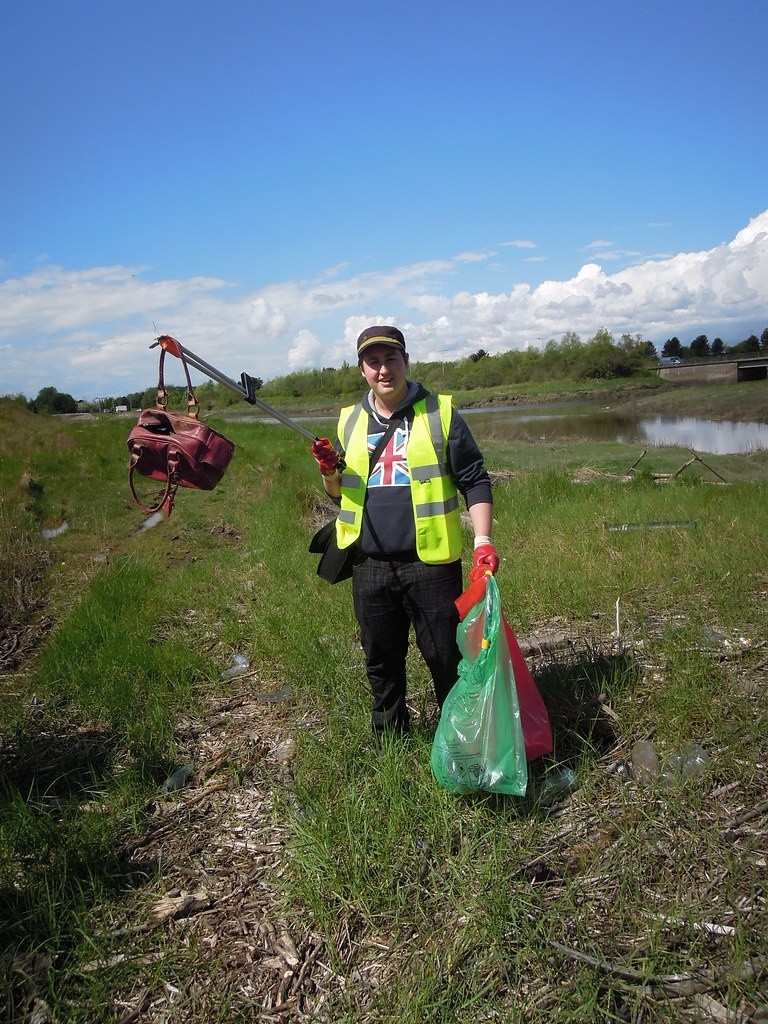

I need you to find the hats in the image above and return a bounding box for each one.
[357,326,405,360]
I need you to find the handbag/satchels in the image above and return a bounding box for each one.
[309,518,362,585]
[126,335,235,513]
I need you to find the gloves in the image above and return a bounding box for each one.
[472,535,500,575]
[311,438,341,482]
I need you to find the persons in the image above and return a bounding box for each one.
[312,326,498,752]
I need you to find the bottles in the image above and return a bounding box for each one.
[219,652,253,681]
[532,769,580,801]
[629,742,664,789]
[273,739,297,766]
[657,744,710,787]
